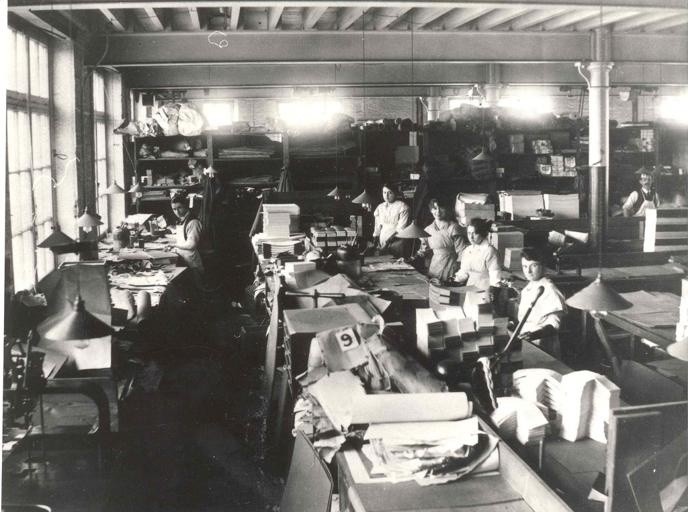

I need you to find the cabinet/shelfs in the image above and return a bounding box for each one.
[421,126,661,206]
[288,123,422,239]
[129,127,287,240]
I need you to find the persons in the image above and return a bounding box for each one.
[514,249,570,362]
[371,179,410,253]
[405,194,468,285]
[621,169,662,217]
[161,191,209,277]
[449,216,509,317]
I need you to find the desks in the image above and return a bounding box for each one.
[3,201,688,511]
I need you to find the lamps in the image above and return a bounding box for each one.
[31,0,120,253]
[326,14,348,200]
[395,10,434,241]
[349,9,381,213]
[561,12,638,322]
[40,0,122,354]
